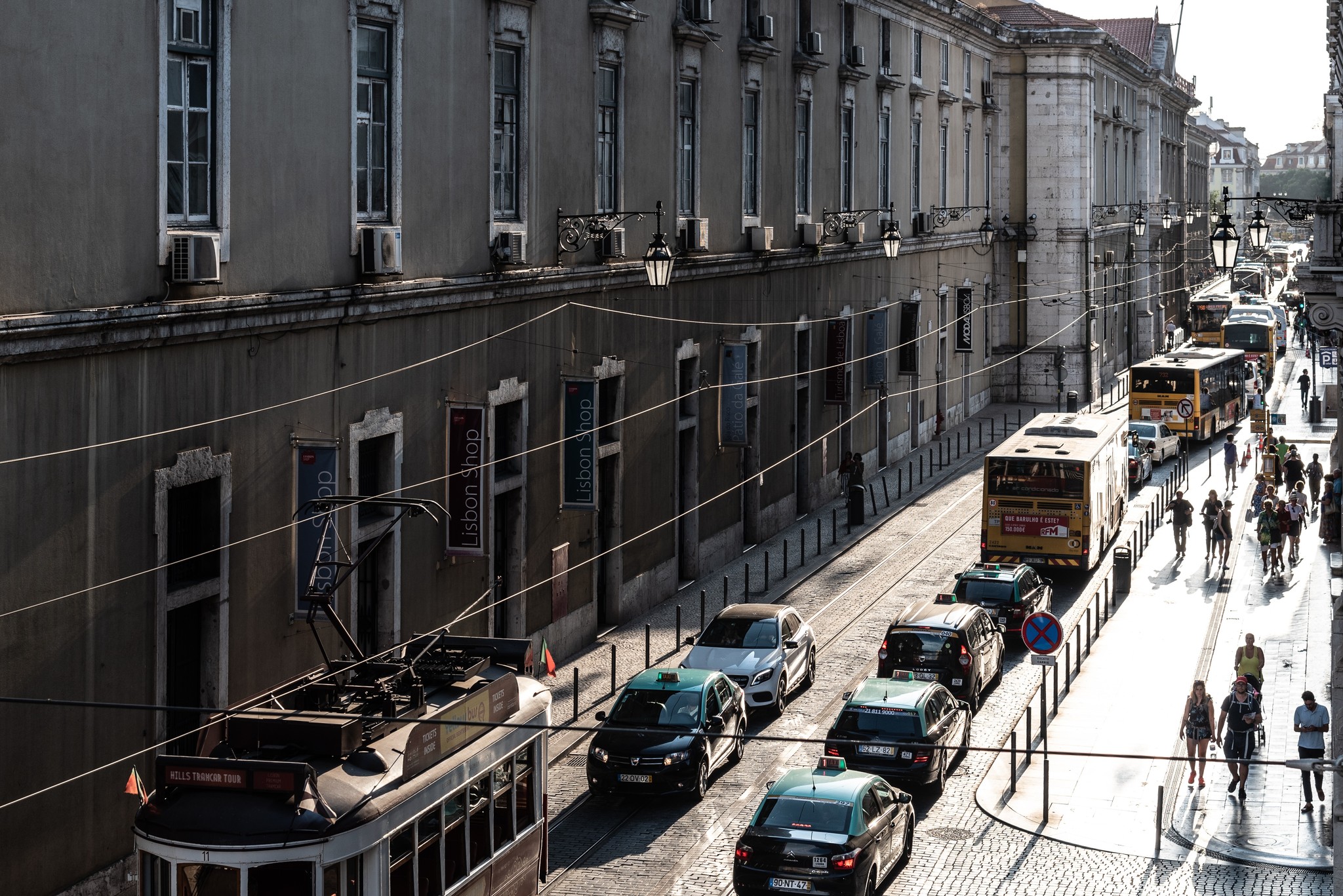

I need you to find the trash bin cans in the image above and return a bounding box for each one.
[1309,396,1321,423]
[1113,545,1131,593]
[848,485,864,524]
[1067,391,1078,413]
[1188,319,1191,335]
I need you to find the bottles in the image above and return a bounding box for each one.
[1210,741,1216,759]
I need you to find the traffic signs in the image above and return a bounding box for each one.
[1319,346,1338,368]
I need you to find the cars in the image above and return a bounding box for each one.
[1128,438,1154,488]
[823,670,972,791]
[587,668,748,799]
[678,602,817,717]
[731,756,916,896]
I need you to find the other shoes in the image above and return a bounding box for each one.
[1238,787,1246,799]
[1301,804,1314,812]
[1317,788,1325,801]
[1228,775,1240,793]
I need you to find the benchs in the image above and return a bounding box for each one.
[1331,578,1343,603]
[1330,552,1343,576]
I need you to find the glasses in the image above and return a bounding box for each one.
[1265,504,1271,507]
[1292,507,1295,513]
[1279,503,1285,506]
[1245,637,1253,641]
[1304,700,1314,707]
[1256,477,1262,479]
[1236,682,1247,687]
[1194,680,1204,685]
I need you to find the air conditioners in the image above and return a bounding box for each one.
[984,80,994,98]
[1115,106,1122,118]
[602,228,625,257]
[1094,255,1101,268]
[917,212,932,234]
[806,32,821,53]
[686,218,708,250]
[1090,305,1099,319]
[751,223,865,250]
[850,45,864,65]
[361,226,402,274]
[499,231,527,263]
[692,0,712,22]
[1106,251,1114,264]
[757,15,773,38]
[172,234,220,283]
[1130,243,1135,258]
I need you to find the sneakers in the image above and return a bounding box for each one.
[1199,777,1205,786]
[1188,770,1196,784]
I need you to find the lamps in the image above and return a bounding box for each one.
[557,200,676,292]
[1092,186,1327,273]
[930,200,995,248]
[822,201,903,261]
[1001,213,1038,251]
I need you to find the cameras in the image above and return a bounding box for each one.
[1166,519,1172,524]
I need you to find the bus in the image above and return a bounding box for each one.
[1216,304,1282,382]
[1186,291,1241,348]
[980,413,1141,573]
[1228,235,1300,327]
[134,495,551,893]
[1125,347,1254,442]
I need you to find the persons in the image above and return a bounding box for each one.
[1223,433,1239,490]
[1188,265,1215,290]
[1165,491,1194,556]
[1166,320,1176,348]
[1216,500,1235,569]
[1234,633,1264,689]
[1297,369,1310,408]
[1216,676,1263,798]
[837,451,867,503]
[1252,687,1259,700]
[1180,680,1216,786]
[1252,388,1268,423]
[1250,427,1342,574]
[1293,313,1338,348]
[1199,387,1217,412]
[1201,490,1223,559]
[1294,691,1330,812]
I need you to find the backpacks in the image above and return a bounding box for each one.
[1212,511,1231,534]
[1228,682,1253,713]
[1187,694,1211,730]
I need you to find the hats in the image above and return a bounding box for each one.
[1289,449,1298,456]
[1289,494,1299,501]
[1236,676,1247,684]
[1222,500,1235,509]
[1253,687,1260,695]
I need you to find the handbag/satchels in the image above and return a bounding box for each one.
[1202,513,1210,519]
[1184,500,1192,527]
[1251,505,1256,518]
[1269,527,1282,544]
[1245,505,1253,523]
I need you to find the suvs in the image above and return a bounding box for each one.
[871,592,1007,713]
[1126,421,1181,464]
[952,562,1053,638]
[1243,361,1264,402]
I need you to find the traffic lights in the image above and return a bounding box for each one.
[1260,394,1265,405]
[1300,320,1303,326]
[1258,354,1266,375]
[1299,295,1305,309]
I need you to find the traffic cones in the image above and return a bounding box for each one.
[1257,433,1264,450]
[1245,443,1253,459]
[1239,450,1251,468]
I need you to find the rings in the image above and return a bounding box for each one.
[1248,722,1249,722]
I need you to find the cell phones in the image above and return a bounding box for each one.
[1304,726,1310,728]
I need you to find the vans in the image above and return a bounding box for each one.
[1259,303,1290,353]
